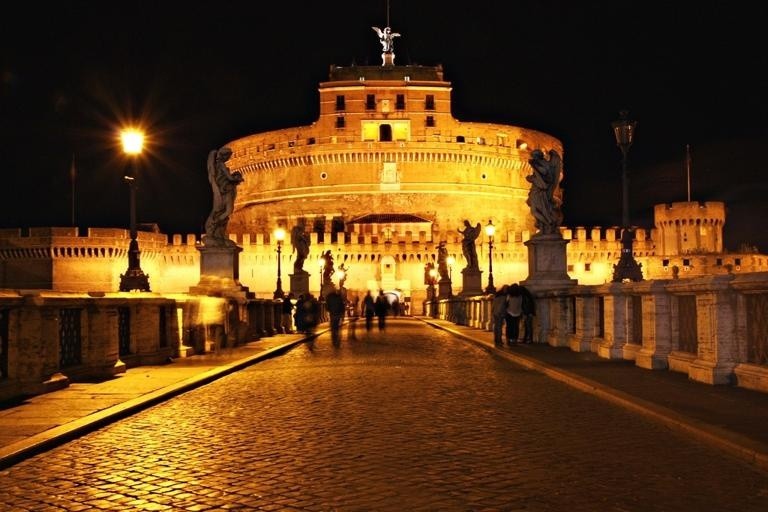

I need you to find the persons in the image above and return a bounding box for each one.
[360,296,409,318]
[435,240,453,281]
[457,220,483,273]
[200,146,245,249]
[319,249,335,284]
[289,222,312,274]
[281,291,346,343]
[422,262,436,284]
[374,287,393,333]
[488,282,537,347]
[520,149,566,234]
[362,289,376,332]
[337,263,351,289]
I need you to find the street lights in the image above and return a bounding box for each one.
[484,217,498,293]
[447,255,456,281]
[271,224,288,298]
[609,108,644,281]
[114,123,159,292]
[317,257,326,289]
[429,268,437,298]
[336,270,344,291]
